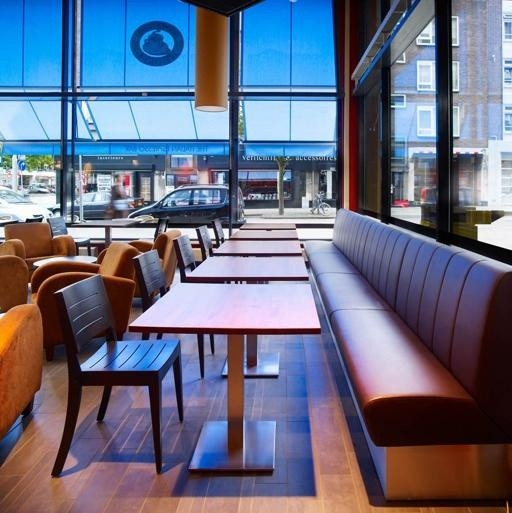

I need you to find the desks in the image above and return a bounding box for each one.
[121,215,325,483]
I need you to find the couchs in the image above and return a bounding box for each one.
[0,213,188,446]
[303,203,511,506]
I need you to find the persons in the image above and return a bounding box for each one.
[103,173,130,218]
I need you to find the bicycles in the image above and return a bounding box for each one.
[311,190,332,215]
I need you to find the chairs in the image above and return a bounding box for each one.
[48,215,247,478]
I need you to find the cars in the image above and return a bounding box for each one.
[48,190,145,221]
[0,182,55,192]
[0,186,54,238]
[419,185,473,206]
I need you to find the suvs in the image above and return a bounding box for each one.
[241,185,287,198]
[128,183,247,229]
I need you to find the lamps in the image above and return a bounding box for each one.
[193,5,233,115]
